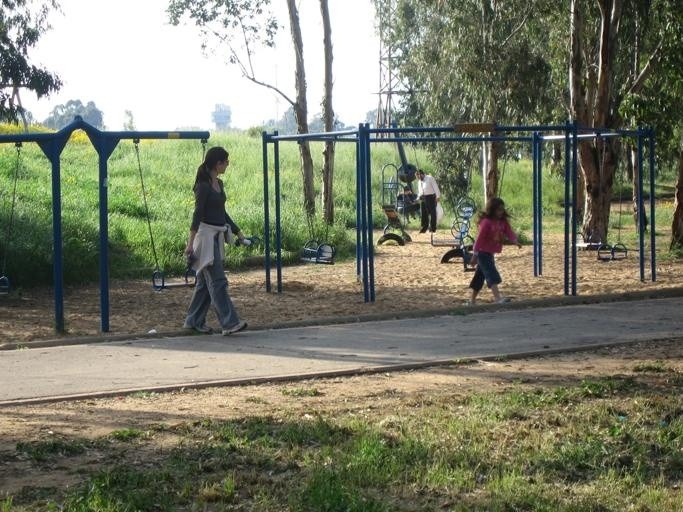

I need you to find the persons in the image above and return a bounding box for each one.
[179,145,248,337]
[412,167,441,234]
[466,197,522,306]
[396,186,418,225]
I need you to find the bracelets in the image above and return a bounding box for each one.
[238,232,243,239]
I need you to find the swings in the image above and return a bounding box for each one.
[132,137,207,289]
[594,134,628,261]
[410,139,481,247]
[0,143,22,296]
[437,139,507,273]
[298,139,337,265]
[571,136,608,251]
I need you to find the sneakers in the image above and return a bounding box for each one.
[184,318,212,333]
[222,321,247,336]
[466,297,511,307]
